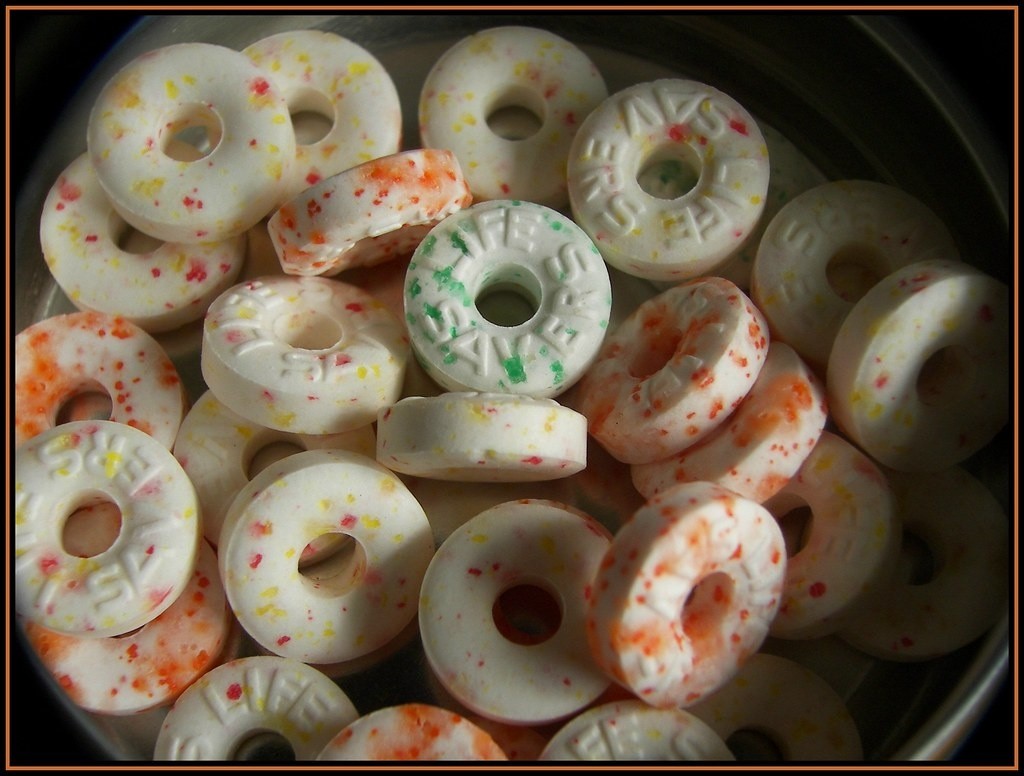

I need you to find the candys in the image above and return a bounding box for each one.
[13,20,1015,761]
